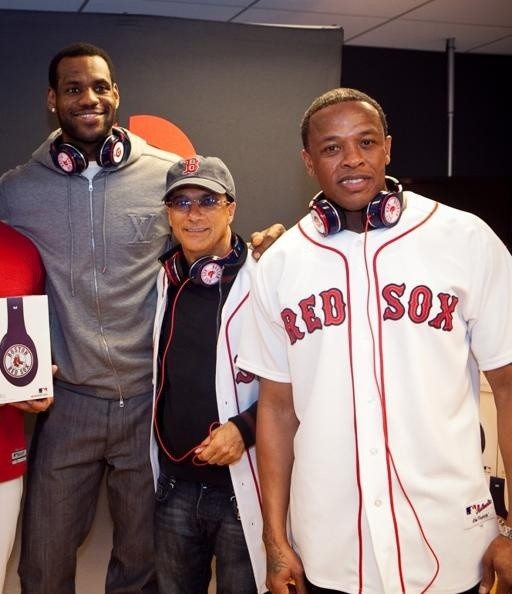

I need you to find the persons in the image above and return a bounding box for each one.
[145,155,258,594]
[1,41,285,594]
[235,87,511,593]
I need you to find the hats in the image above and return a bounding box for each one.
[161,156,237,203]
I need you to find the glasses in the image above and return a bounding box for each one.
[167,195,223,209]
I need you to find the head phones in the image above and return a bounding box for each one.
[164,234,246,288]
[47,126,131,175]
[309,176,404,237]
[0,297,38,387]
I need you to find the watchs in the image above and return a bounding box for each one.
[497,525,512,540]
